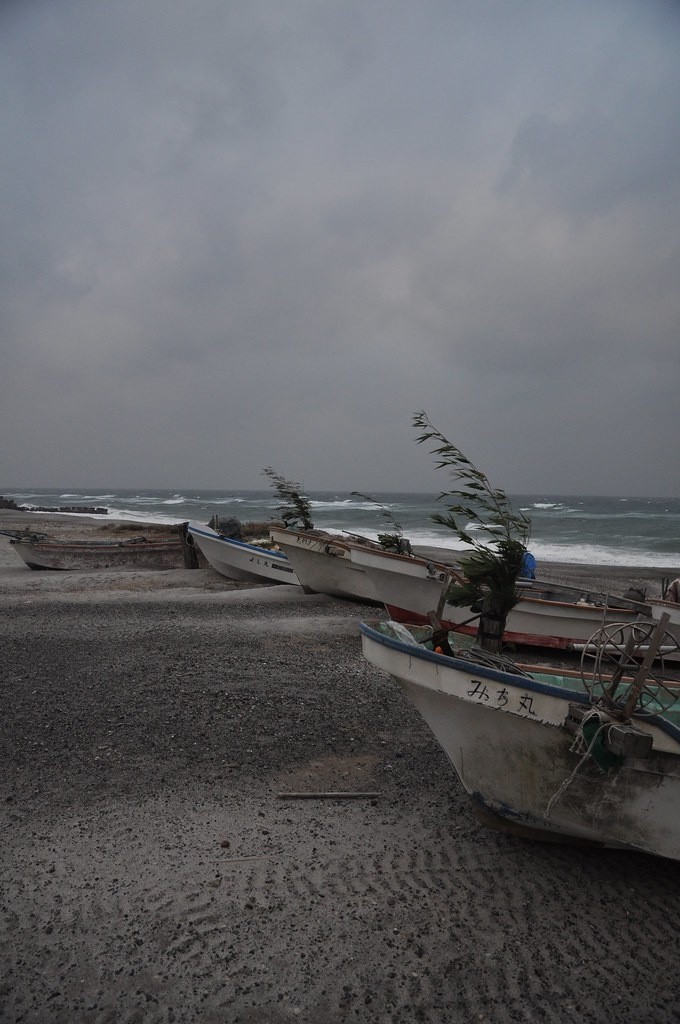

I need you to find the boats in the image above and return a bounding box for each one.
[358,593,680,863]
[0,521,200,570]
[269,526,680,663]
[188,521,301,586]
